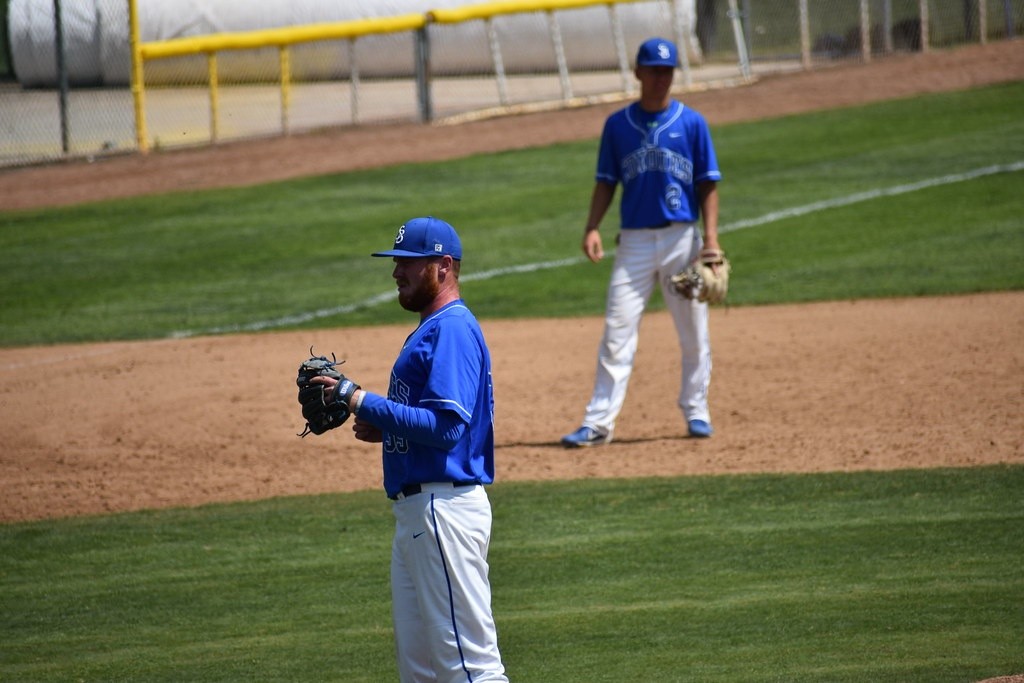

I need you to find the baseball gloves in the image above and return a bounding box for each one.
[296,344,361,438]
[665,247,733,306]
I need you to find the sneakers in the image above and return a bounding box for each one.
[687,419,712,435]
[562,427,610,446]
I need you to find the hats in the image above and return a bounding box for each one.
[637,37,679,68]
[374,216,464,262]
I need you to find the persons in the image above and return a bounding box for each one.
[296,217,512,682]
[559,38,733,449]
